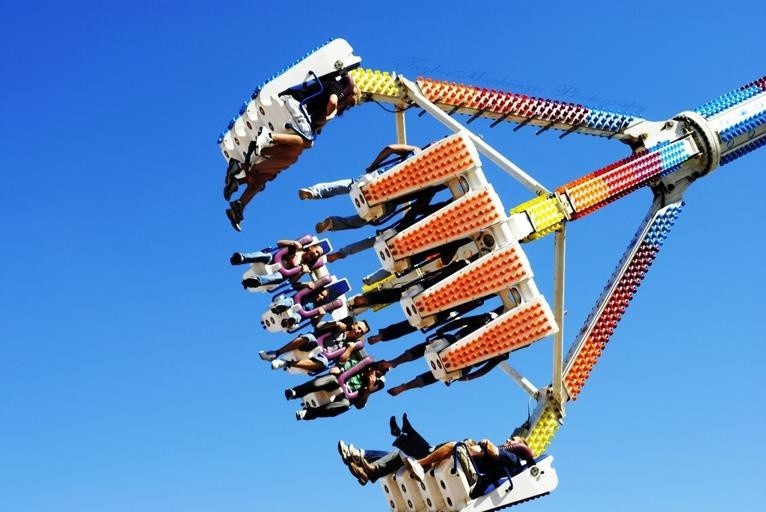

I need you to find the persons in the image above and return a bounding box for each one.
[224,60,390,232]
[300,143,510,396]
[339,412,529,487]
[231,240,379,420]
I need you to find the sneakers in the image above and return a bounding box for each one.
[296,409,307,420]
[242,278,260,287]
[285,389,296,400]
[260,350,276,361]
[271,305,285,314]
[230,252,244,265]
[298,189,314,200]
[316,217,332,234]
[272,359,285,369]
[327,251,343,263]
[281,317,296,328]
[227,199,244,232]
[339,440,376,485]
[224,158,240,201]
[363,277,369,285]
